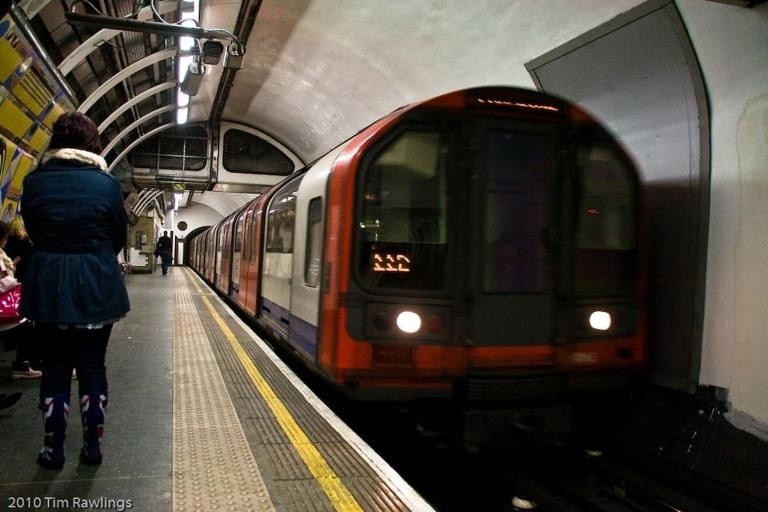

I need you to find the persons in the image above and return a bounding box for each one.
[0,112,130,469]
[154,231,171,275]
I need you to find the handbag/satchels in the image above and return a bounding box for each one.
[0,284,22,320]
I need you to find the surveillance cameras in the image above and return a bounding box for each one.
[223,38,247,72]
[202,39,223,63]
[181,62,204,95]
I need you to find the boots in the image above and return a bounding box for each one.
[38,396,69,470]
[80,392,108,465]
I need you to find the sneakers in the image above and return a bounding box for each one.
[10,366,43,381]
[0,391,23,412]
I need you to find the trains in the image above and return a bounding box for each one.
[185,84,646,451]
